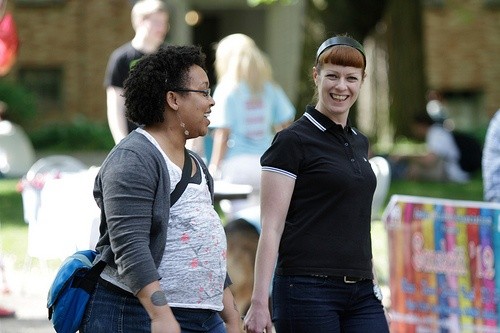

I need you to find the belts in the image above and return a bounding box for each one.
[340,276,359,285]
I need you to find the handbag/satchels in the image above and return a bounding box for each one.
[47,250,98,333]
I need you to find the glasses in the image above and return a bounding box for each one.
[167,89,211,98]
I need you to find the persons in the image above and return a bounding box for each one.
[105,1,173,148]
[246,37,389,333]
[386,103,470,184]
[481,109,500,210]
[48,49,243,333]
[207,33,298,232]
